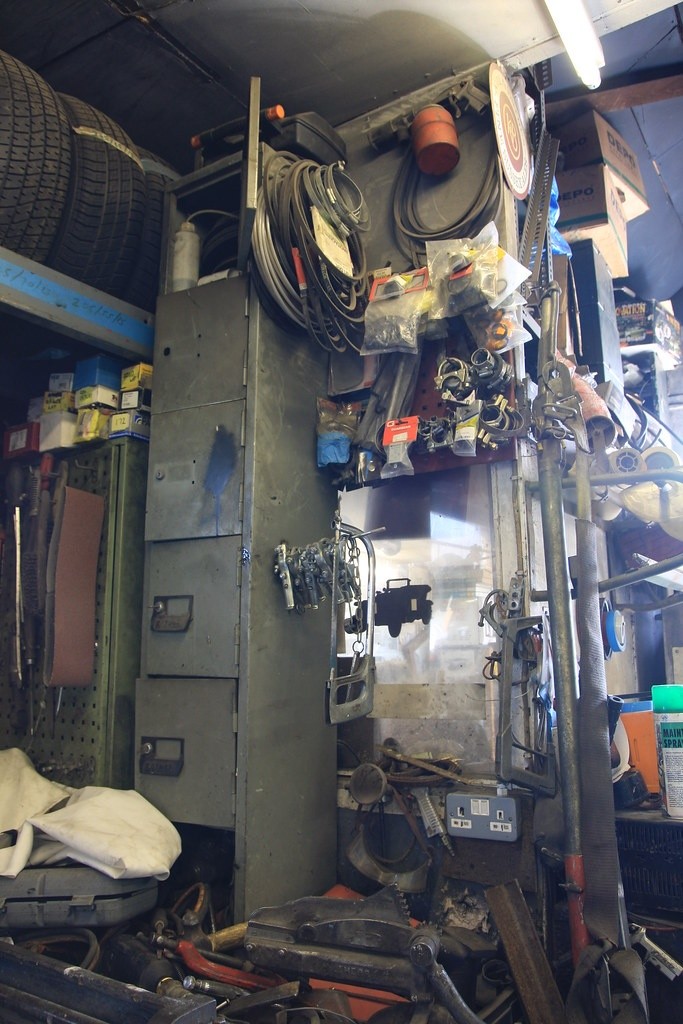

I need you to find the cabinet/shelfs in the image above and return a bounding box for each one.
[127,266,339,935]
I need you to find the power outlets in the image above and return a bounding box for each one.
[445,794,523,844]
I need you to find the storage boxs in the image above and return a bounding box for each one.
[4,334,152,462]
[0,858,160,934]
[615,685,683,921]
[553,108,683,369]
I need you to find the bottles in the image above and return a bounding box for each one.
[197,267,240,287]
[172,222,201,291]
[410,103,459,175]
[651,683,683,820]
